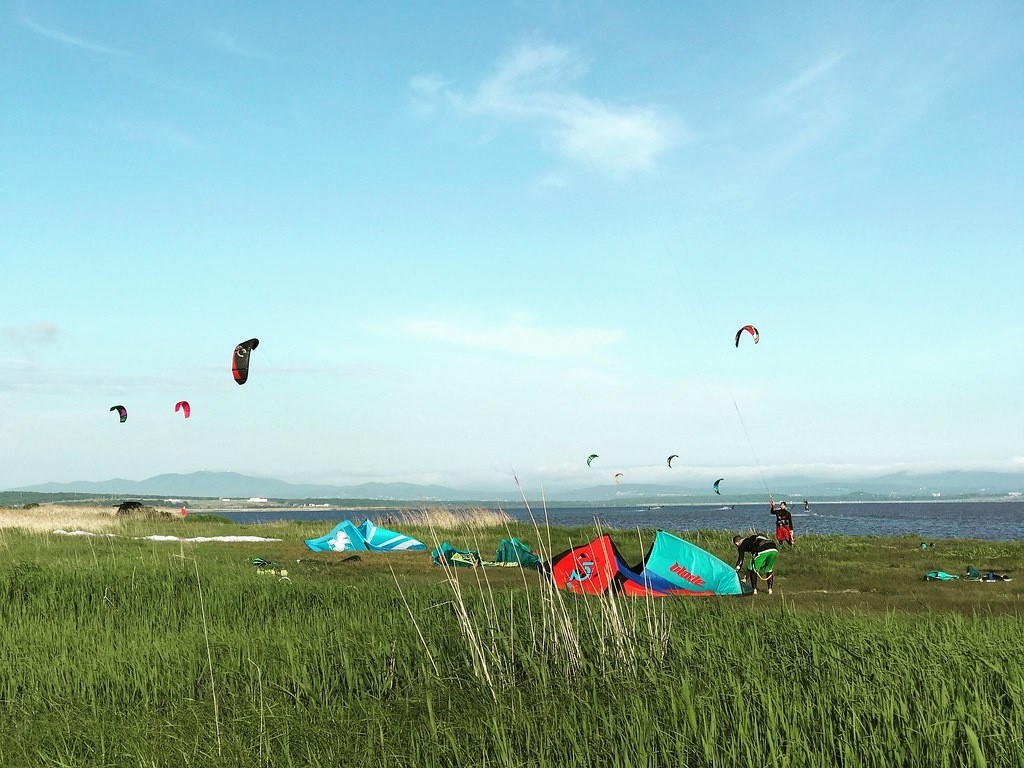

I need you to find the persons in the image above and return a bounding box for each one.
[804,501,809,511]
[770,501,793,547]
[733,534,779,594]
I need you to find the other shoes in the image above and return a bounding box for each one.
[768,589,773,595]
[754,589,757,594]
